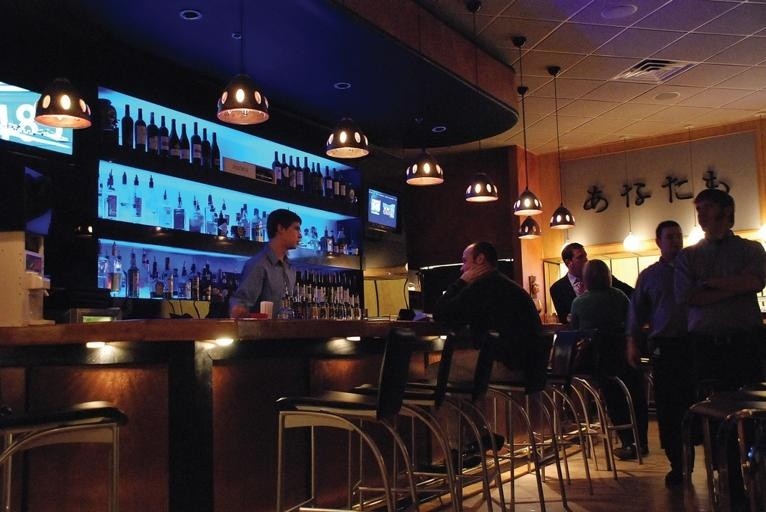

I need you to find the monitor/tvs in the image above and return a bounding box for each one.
[364,186,402,230]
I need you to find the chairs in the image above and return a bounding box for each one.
[0,401,128,512]
[690,374,765,511]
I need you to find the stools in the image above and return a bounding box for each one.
[482,333,567,512]
[349,330,461,512]
[621,345,660,456]
[274,328,418,512]
[572,328,643,492]
[402,333,504,512]
[522,329,594,512]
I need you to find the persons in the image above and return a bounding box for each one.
[621,221,705,490]
[554,259,634,447]
[670,187,766,512]
[432,238,547,469]
[549,242,650,460]
[228,209,303,316]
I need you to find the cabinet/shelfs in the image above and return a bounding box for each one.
[45,141,365,319]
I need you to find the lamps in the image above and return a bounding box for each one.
[512,34,542,215]
[327,32,370,160]
[465,2,498,204]
[35,68,92,132]
[515,86,543,240]
[545,64,574,229]
[217,19,270,125]
[406,22,445,186]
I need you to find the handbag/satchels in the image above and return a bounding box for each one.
[567,337,595,371]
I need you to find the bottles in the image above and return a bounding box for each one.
[98,98,361,322]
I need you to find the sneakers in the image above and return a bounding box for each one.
[462,428,505,469]
[666,471,682,485]
[615,444,649,457]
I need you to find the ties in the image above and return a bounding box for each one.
[577,281,583,296]
